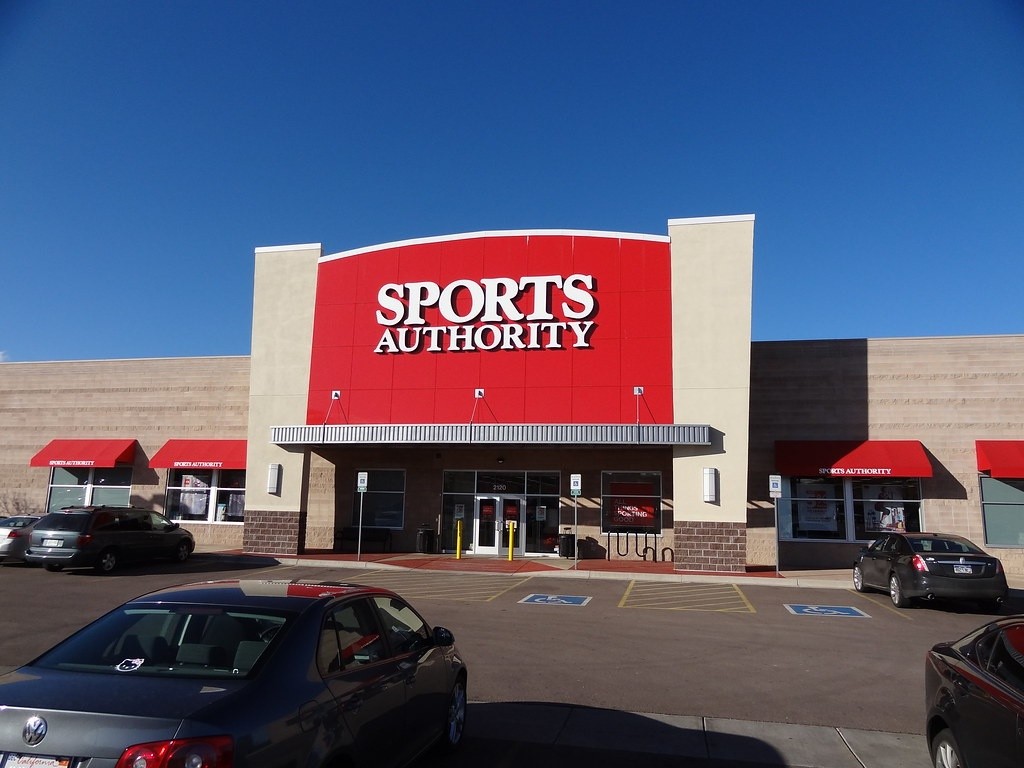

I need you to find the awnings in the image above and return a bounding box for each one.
[975,440,1024,479]
[774,440,934,477]
[30,439,137,468]
[148,440,247,469]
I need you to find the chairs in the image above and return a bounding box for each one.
[233,641,270,672]
[199,616,261,663]
[9,521,25,527]
[932,541,963,551]
[894,541,924,551]
[115,635,168,663]
[175,644,221,670]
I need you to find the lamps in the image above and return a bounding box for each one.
[497,456,505,464]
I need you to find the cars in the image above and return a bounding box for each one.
[0,576,468,768]
[922,614,1024,768]
[853,531,1010,613]
[23,505,196,576]
[0,515,44,564]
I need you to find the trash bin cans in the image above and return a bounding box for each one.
[559,534,575,557]
[417,529,436,553]
